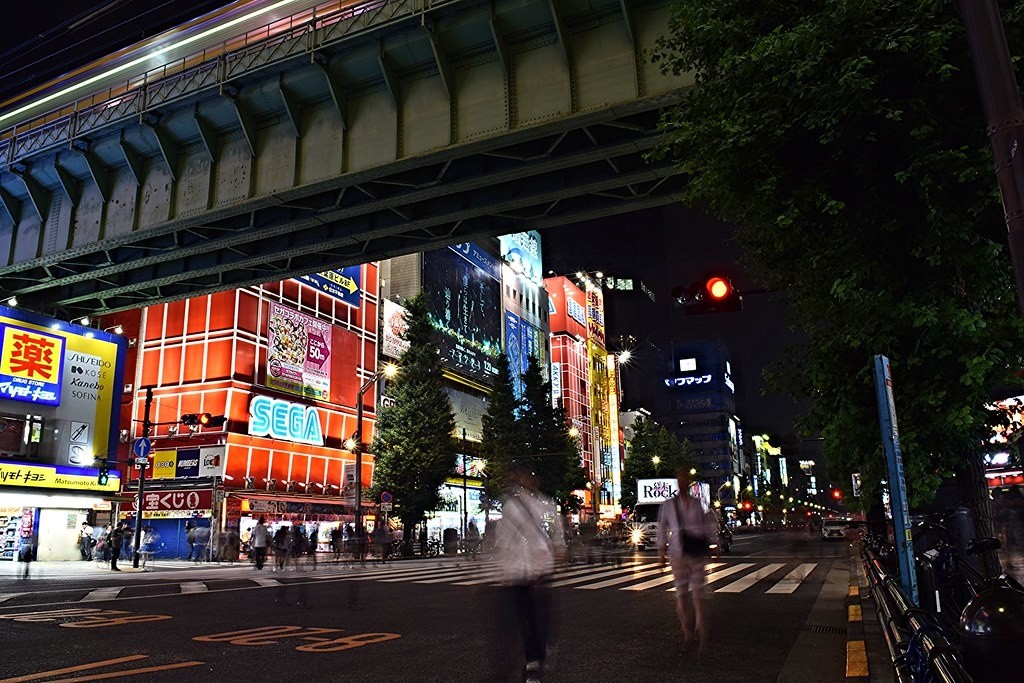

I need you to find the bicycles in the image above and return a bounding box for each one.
[899,498,1024,641]
[853,507,897,599]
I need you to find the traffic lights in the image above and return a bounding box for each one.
[833,489,842,499]
[671,271,744,315]
[744,502,751,510]
[98,466,112,486]
[181,413,211,426]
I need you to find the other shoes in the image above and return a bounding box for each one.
[111,567,121,572]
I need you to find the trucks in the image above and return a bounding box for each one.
[629,478,711,551]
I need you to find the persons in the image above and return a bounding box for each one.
[992,484,1024,555]
[186,523,240,562]
[468,522,479,539]
[249,517,318,571]
[77,520,146,562]
[655,471,713,651]
[330,522,403,557]
[496,462,566,683]
[110,521,125,571]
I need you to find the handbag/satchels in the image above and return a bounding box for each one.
[90,538,96,548]
[97,541,104,550]
[681,529,709,557]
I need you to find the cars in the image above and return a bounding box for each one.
[821,519,850,541]
[705,519,733,556]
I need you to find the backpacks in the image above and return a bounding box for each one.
[106,533,112,547]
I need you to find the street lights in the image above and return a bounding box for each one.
[740,485,753,503]
[760,491,771,501]
[342,362,399,559]
[718,481,731,501]
